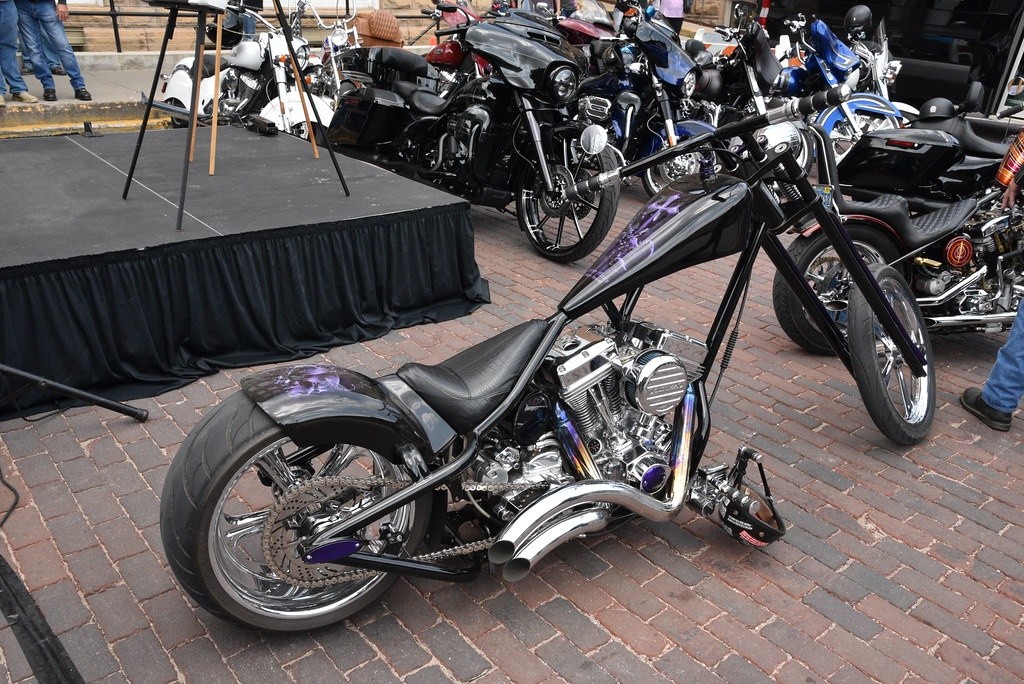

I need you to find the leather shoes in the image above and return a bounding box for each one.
[42,89,57,101]
[959,386,1013,431]
[75,88,92,100]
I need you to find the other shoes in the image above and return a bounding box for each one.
[21,66,35,75]
[51,66,67,76]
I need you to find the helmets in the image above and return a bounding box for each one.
[844,4,873,32]
[919,97,957,120]
[206,9,244,48]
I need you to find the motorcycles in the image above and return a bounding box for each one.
[139,1,1024,355]
[160,84,936,640]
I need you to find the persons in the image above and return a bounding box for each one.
[0,0,92,107]
[492,0,686,36]
[960,163,1024,430]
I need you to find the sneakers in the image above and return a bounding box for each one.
[12,91,38,103]
[0,95,7,107]
